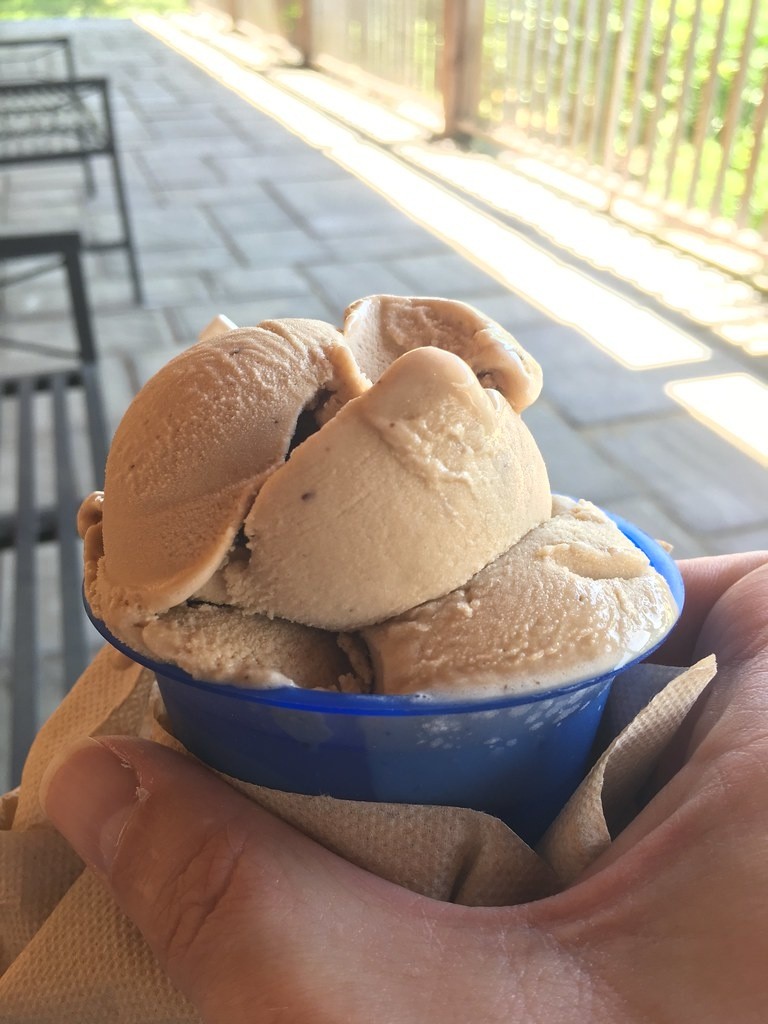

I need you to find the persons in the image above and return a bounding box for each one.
[38,551,768,1024]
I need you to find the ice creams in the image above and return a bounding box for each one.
[74,293,678,703]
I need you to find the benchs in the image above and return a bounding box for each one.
[0,33,148,305]
[0,227,115,789]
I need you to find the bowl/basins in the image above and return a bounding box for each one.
[82,492,687,849]
